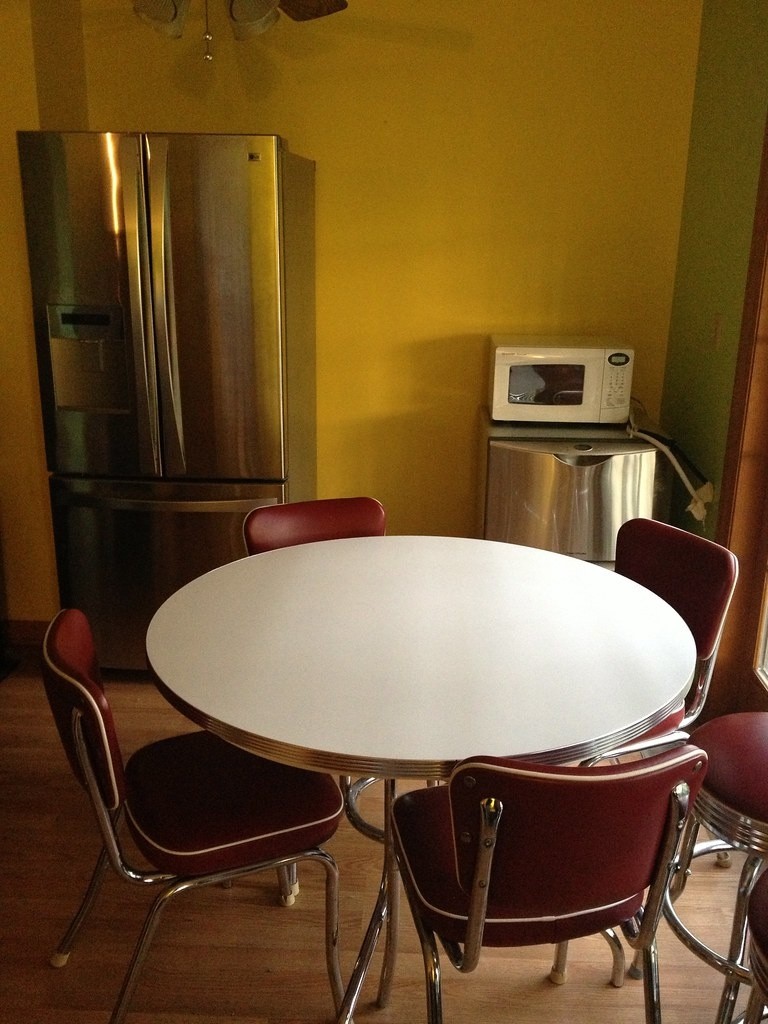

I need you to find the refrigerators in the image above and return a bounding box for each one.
[15,129,316,674]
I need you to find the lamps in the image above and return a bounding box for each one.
[132,0,280,41]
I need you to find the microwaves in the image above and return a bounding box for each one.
[484,333,636,425]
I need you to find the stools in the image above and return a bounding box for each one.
[743,868,768,1024]
[629,712,768,1024]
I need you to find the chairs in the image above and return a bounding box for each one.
[41,495,739,1024]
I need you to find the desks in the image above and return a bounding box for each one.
[144,536,698,1024]
